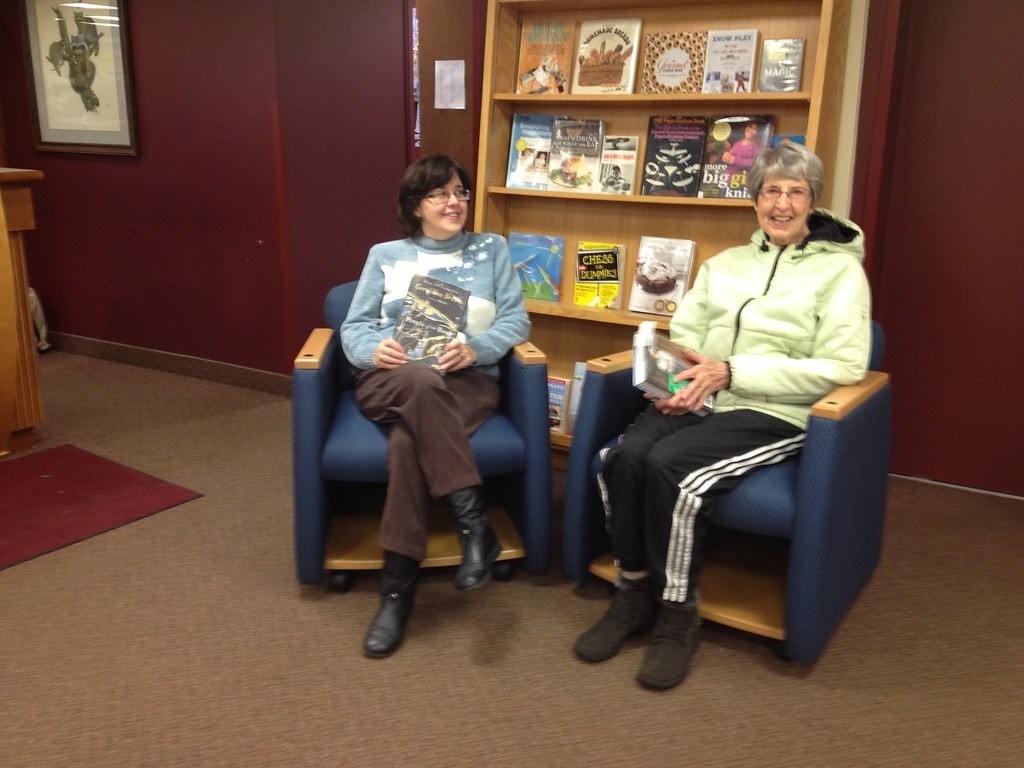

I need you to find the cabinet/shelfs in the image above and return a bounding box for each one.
[472,0,884,512]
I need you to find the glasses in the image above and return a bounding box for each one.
[422,188,470,204]
[758,186,812,201]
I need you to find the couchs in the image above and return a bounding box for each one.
[565,319,894,664]
[292,279,553,594]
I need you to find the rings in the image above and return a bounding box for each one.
[698,385,704,391]
[458,354,463,358]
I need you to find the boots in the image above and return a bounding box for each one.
[574,569,662,664]
[637,588,701,689]
[450,486,502,593]
[362,548,422,660]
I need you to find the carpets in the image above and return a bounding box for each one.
[0,443,204,572]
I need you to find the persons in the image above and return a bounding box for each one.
[720,121,762,198]
[573,140,874,693]
[601,166,630,191]
[339,154,531,661]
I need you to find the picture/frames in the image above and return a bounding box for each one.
[12,0,142,162]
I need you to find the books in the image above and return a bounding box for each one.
[547,376,570,435]
[515,18,577,95]
[771,135,805,151]
[570,18,643,96]
[699,113,778,199]
[629,234,697,317]
[391,274,471,376]
[505,113,710,197]
[507,231,565,301]
[632,330,714,417]
[640,31,708,95]
[700,29,759,94]
[757,37,807,93]
[573,240,627,309]
